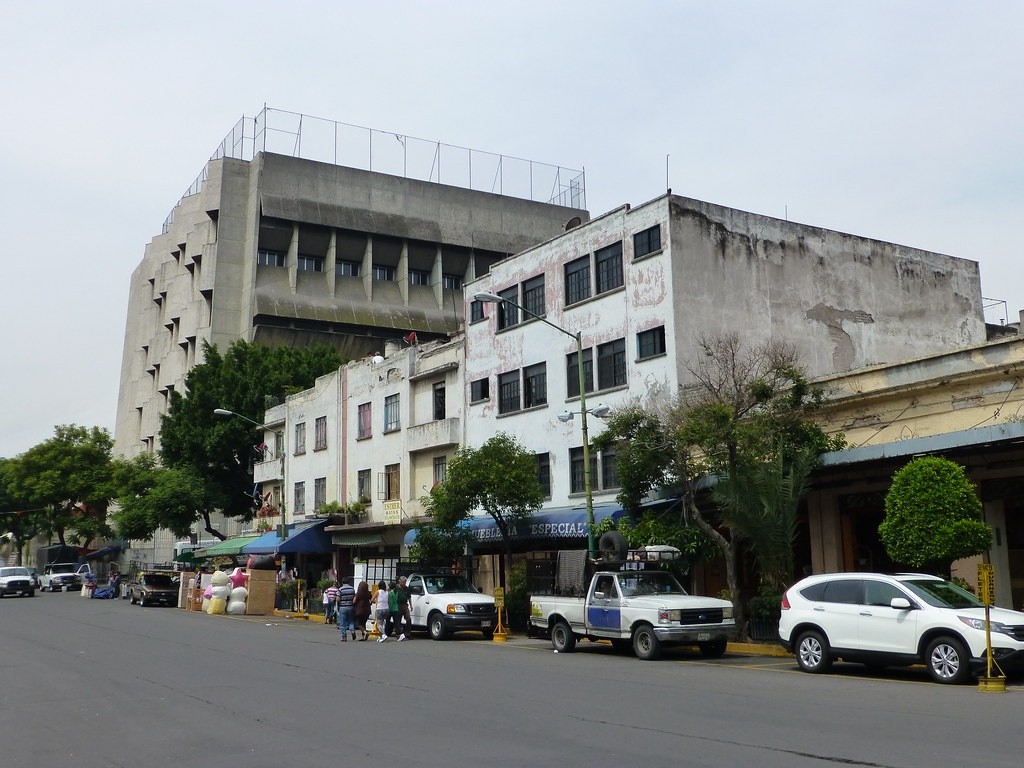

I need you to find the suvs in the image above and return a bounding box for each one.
[0,566,34,598]
[777,572,1024,685]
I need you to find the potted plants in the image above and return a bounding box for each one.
[256,520,272,532]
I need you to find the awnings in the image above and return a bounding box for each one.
[404,498,685,549]
[194,542,222,558]
[242,519,334,554]
[207,537,260,556]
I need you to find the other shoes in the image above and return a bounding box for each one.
[364,632,370,641]
[340,637,347,642]
[358,639,364,641]
[351,631,356,640]
[396,633,405,642]
[406,636,414,640]
[325,622,332,624]
[377,634,388,643]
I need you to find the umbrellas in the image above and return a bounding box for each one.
[173,552,205,563]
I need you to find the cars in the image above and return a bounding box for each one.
[26,567,38,588]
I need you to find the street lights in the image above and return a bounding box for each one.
[214,408,287,582]
[474,292,609,559]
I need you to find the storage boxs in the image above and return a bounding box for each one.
[245,580,277,615]
[201,573,212,589]
[246,569,277,581]
[180,572,196,588]
[178,587,189,608]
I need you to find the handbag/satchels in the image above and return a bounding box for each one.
[322,592,330,604]
[371,616,381,634]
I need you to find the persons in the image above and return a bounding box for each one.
[371,580,389,642]
[367,352,372,357]
[353,582,373,641]
[396,576,413,640]
[373,351,384,363]
[335,577,356,642]
[106,570,125,599]
[323,582,337,623]
[385,582,405,641]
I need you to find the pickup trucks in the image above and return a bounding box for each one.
[37,563,90,592]
[529,571,737,660]
[129,574,179,607]
[368,573,497,639]
